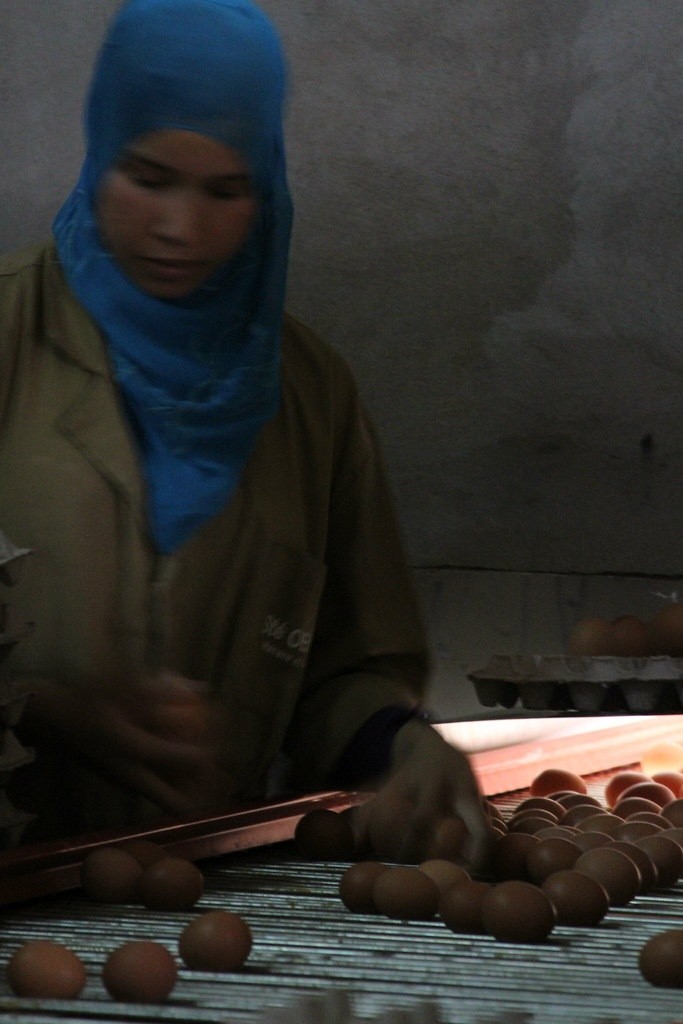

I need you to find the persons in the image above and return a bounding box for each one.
[2,0,494,875]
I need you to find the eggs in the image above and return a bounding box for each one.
[2,600,683,998]
